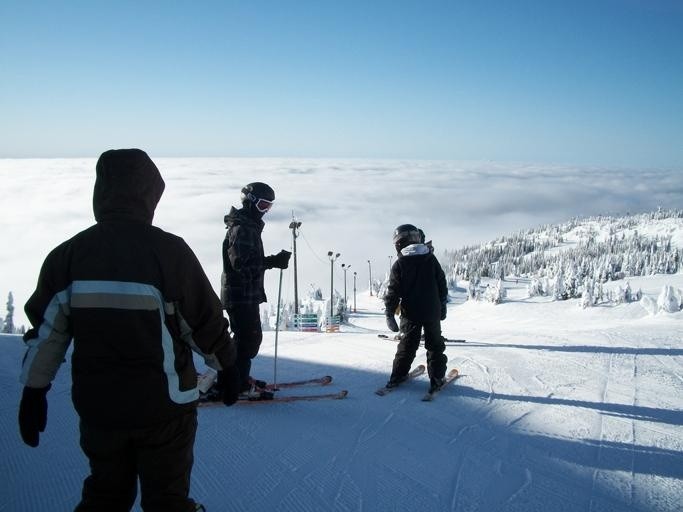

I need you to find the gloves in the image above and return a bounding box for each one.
[20,384,51,448]
[387,318,399,333]
[270,252,289,269]
[441,305,447,320]
[218,368,239,408]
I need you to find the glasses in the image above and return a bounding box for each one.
[242,188,274,213]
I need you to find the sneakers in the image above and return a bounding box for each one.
[239,376,274,399]
[390,374,447,390]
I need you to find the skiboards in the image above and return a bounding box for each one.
[378,334,465,347]
[374,364,458,403]
[195,376,349,407]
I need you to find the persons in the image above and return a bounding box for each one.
[17,145,240,512]
[211,178,291,401]
[382,222,450,394]
[397,228,435,340]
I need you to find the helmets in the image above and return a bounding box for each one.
[240,182,275,205]
[392,224,426,249]
